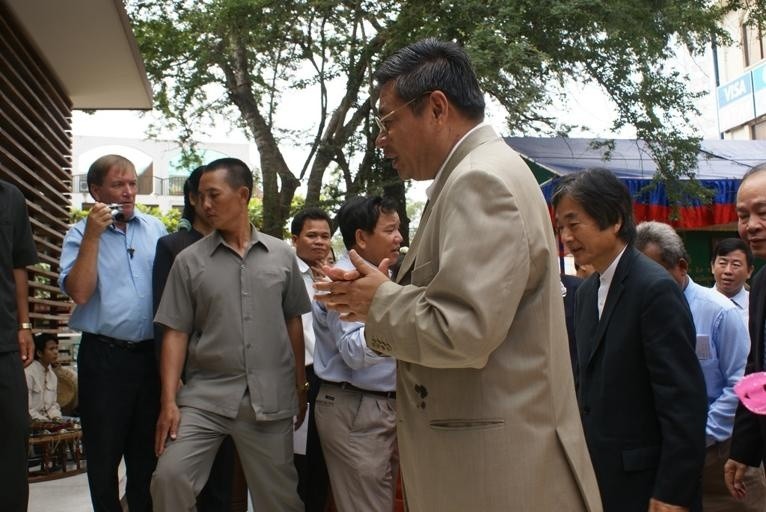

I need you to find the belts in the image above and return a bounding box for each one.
[325,382,396,399]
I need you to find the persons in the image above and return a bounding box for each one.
[56,154,404,512]
[24,333,67,469]
[550,162,766,512]
[0,181,42,512]
[311,37,603,512]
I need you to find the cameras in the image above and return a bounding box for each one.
[105,202,126,226]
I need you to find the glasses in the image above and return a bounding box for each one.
[375,91,432,134]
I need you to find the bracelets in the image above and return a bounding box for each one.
[16,322,33,330]
